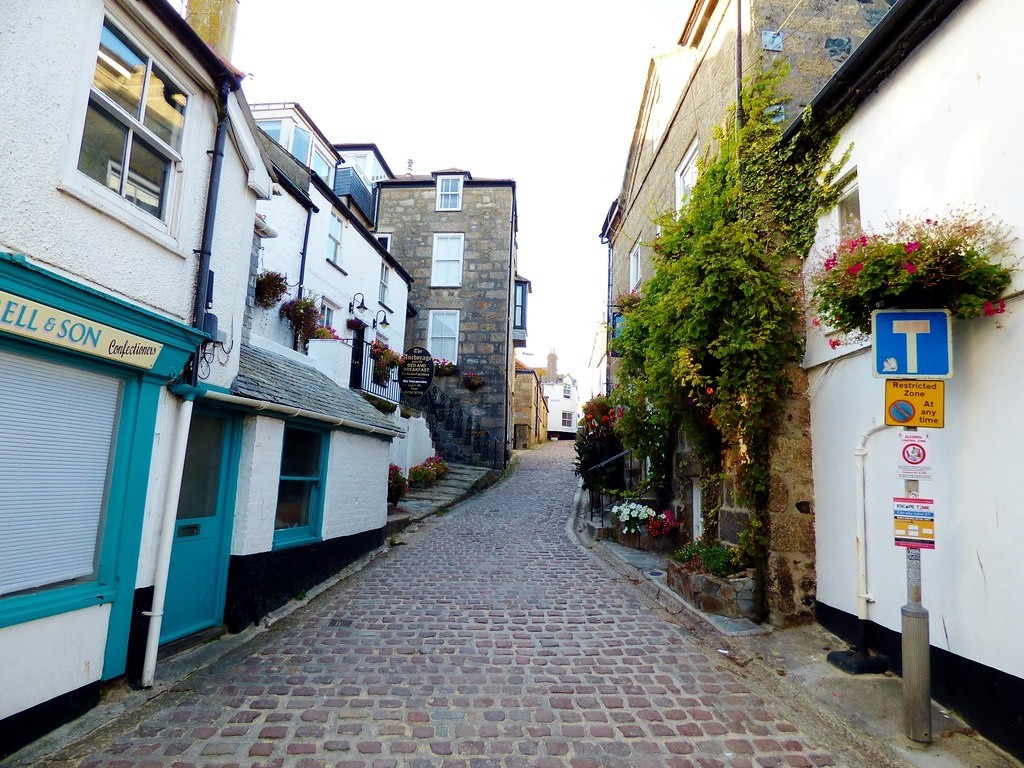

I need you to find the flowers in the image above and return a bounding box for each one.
[277,297,345,354]
[431,357,487,389]
[550,433,560,438]
[254,269,288,309]
[613,286,644,311]
[611,500,711,575]
[370,339,409,384]
[578,380,627,433]
[791,197,1024,349]
[389,455,451,507]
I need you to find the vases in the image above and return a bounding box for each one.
[387,487,403,507]
[409,479,424,488]
[466,381,481,391]
[616,516,655,553]
[550,437,558,441]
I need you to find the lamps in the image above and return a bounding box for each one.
[373,310,390,330]
[349,293,368,314]
[203,313,218,346]
